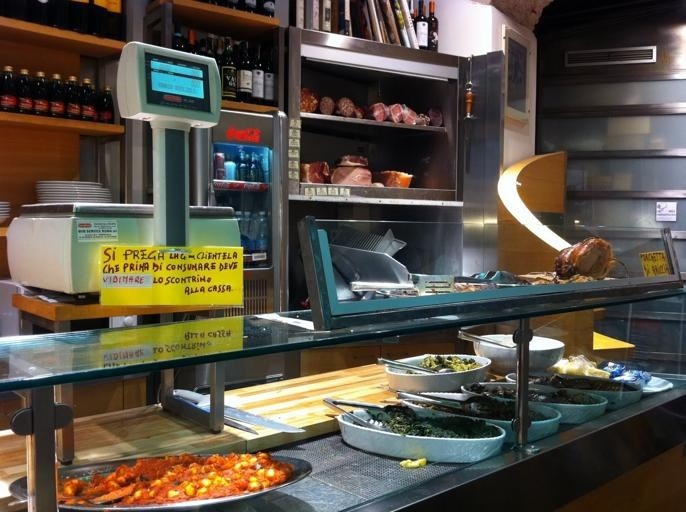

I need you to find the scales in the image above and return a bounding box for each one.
[5,40,242,302]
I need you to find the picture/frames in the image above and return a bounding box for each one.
[501,25,533,123]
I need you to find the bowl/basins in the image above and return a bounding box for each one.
[461,382,609,425]
[386,354,491,392]
[403,392,562,445]
[505,368,641,411]
[473,334,565,376]
[336,409,504,464]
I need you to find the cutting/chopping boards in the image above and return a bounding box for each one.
[0,404,246,512]
[160,348,507,454]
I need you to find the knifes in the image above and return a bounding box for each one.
[174,388,306,434]
[222,419,260,436]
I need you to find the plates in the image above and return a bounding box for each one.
[0,180,113,225]
[10,454,312,510]
[609,369,673,397]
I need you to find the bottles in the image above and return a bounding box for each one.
[213,147,271,265]
[414,0,428,50]
[0,1,278,125]
[429,0,438,51]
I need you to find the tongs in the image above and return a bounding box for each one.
[322,398,392,432]
[457,331,517,348]
[396,391,487,414]
[376,356,457,374]
